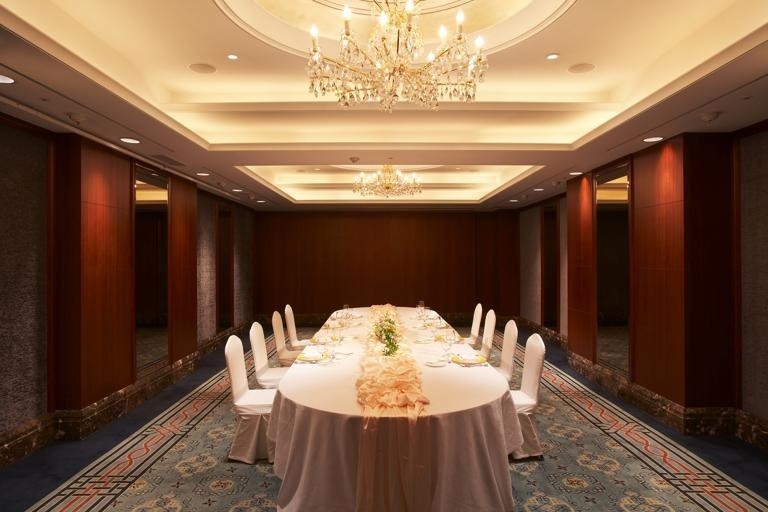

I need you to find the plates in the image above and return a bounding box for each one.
[296,310,352,368]
[418,314,487,364]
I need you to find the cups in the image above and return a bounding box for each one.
[343,305,349,314]
[416,301,430,321]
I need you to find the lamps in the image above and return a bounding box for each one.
[298,1,489,114]
[352,158,423,199]
